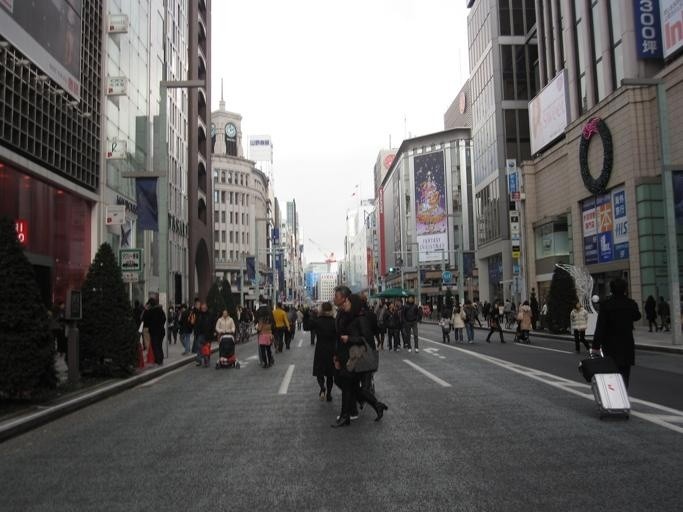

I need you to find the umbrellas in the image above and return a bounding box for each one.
[378,287,417,306]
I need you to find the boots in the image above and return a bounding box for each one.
[353,386,388,421]
[331,390,350,428]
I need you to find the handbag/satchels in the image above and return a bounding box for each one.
[491,323,498,328]
[579,353,619,381]
[517,312,523,320]
[345,346,380,373]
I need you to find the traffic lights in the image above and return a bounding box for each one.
[389,267,402,274]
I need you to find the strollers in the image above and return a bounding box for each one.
[512,319,533,344]
[212,330,242,370]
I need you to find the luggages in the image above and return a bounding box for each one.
[590,346,631,420]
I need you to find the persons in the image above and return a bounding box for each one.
[133,297,166,367]
[439,294,546,345]
[333,286,374,421]
[591,278,641,391]
[372,295,422,353]
[657,295,670,332]
[567,302,591,355]
[423,302,437,320]
[167,297,214,368]
[301,301,337,403]
[235,296,317,369]
[215,309,236,345]
[644,295,659,333]
[330,294,387,429]
